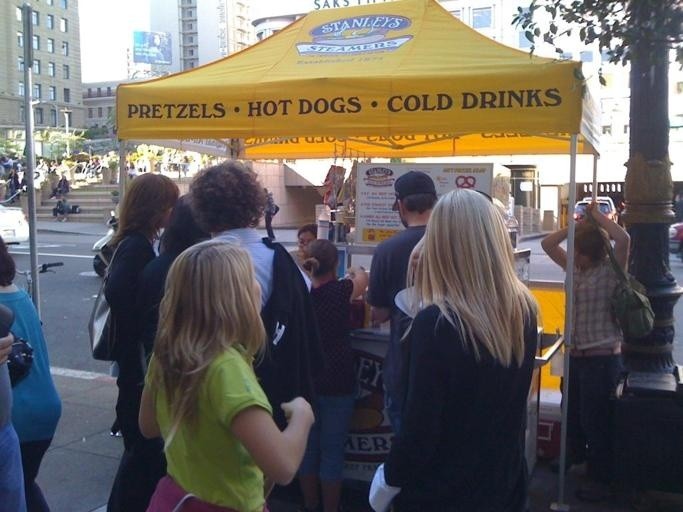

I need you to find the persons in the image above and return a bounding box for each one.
[1,237,62,512]
[542,201,631,500]
[52,198,73,223]
[93,161,539,512]
[0,153,219,206]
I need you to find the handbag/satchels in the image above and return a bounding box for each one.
[85,280,132,361]
[612,282,656,339]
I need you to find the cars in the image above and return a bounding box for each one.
[669,222,683,264]
[574,196,619,223]
[0,204,30,243]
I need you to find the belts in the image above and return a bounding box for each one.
[568,347,622,358]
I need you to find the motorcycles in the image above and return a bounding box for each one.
[93,211,119,278]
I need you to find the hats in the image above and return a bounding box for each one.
[391,171,437,211]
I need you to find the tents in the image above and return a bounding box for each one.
[116,0,606,512]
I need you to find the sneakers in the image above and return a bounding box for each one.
[577,478,611,501]
